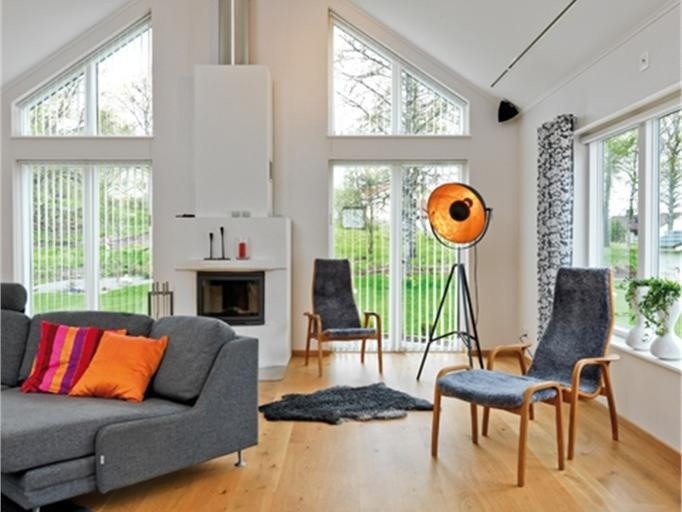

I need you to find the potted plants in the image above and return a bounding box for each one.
[626,277,681,361]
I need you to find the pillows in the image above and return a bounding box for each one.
[20,315,170,401]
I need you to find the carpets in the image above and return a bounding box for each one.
[260,379,435,424]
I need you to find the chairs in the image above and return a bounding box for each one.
[304,256,383,373]
[430,267,623,488]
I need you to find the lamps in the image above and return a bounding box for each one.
[417,181,491,381]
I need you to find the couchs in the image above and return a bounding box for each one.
[0,282,262,507]
